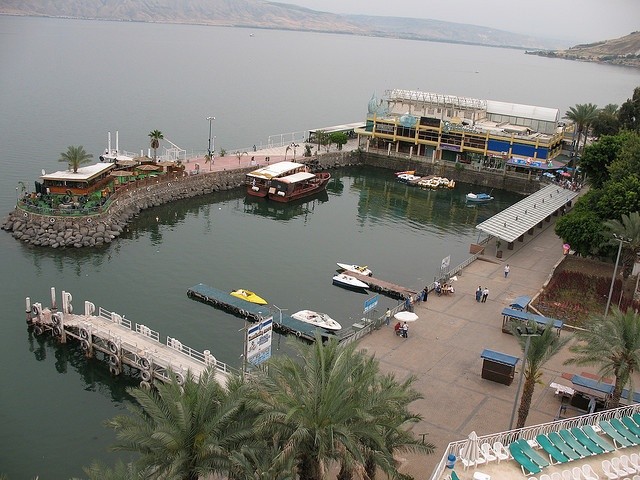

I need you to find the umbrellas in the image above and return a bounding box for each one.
[394,311,418,323]
[136,164,160,176]
[116,160,138,170]
[547,174,555,180]
[556,170,564,176]
[132,157,153,165]
[560,172,571,177]
[543,172,550,175]
[110,170,132,184]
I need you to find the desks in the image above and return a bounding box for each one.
[442,287,448,293]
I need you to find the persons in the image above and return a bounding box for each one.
[504,264,510,278]
[482,287,489,302]
[558,177,581,192]
[394,322,401,335]
[402,322,409,339]
[435,282,454,296]
[475,285,482,302]
[423,286,429,301]
[385,308,391,325]
[407,294,415,313]
[21,185,110,210]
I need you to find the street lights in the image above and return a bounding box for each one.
[604,233,634,323]
[206,116,217,156]
[507,323,546,431]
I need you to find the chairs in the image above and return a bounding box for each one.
[528,477,538,480]
[548,432,582,461]
[460,449,474,469]
[601,422,632,448]
[536,434,567,465]
[633,475,640,479]
[572,467,588,480]
[540,475,550,480]
[516,439,549,469]
[480,443,498,463]
[622,478,631,480]
[561,430,592,457]
[584,426,614,451]
[474,447,486,468]
[451,471,460,480]
[610,458,629,478]
[620,455,637,475]
[626,416,640,439]
[630,453,640,472]
[493,442,510,463]
[509,443,542,476]
[602,460,619,480]
[551,473,563,479]
[612,419,640,445]
[571,428,603,454]
[472,472,491,480]
[562,471,575,480]
[581,465,599,480]
[633,414,640,425]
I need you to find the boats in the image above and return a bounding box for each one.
[332,273,370,292]
[267,171,332,204]
[465,193,495,203]
[291,309,342,330]
[393,170,457,192]
[244,161,312,198]
[336,262,373,277]
[229,289,268,305]
